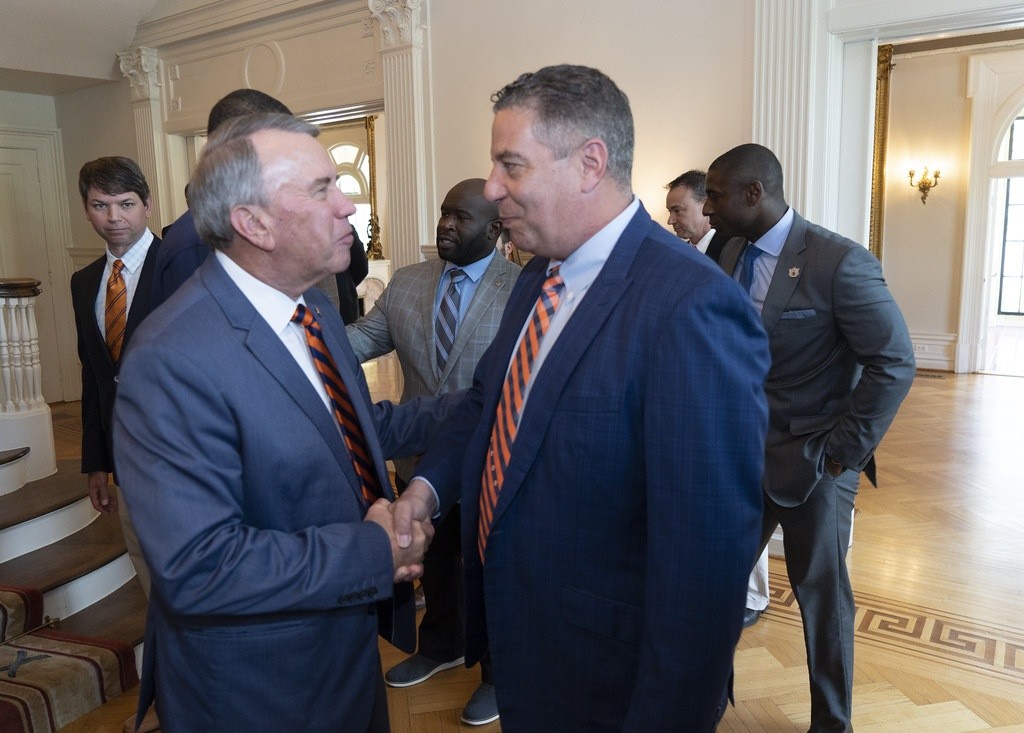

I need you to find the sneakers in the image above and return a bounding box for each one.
[742,605,767,627]
[413,583,426,610]
[121,703,159,733]
[460,680,501,726]
[384,651,466,688]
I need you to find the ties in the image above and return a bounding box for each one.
[434,269,470,372]
[104,260,127,367]
[289,304,385,511]
[477,265,566,567]
[738,245,764,294]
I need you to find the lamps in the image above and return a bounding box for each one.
[908,158,942,205]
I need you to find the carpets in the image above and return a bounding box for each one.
[0,582,141,733]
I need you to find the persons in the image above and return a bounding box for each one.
[69,156,165,732]
[703,142,919,732]
[336,222,368,325]
[665,169,772,628]
[148,87,294,311]
[110,113,469,732]
[344,177,526,726]
[387,62,771,733]
[162,181,192,238]
